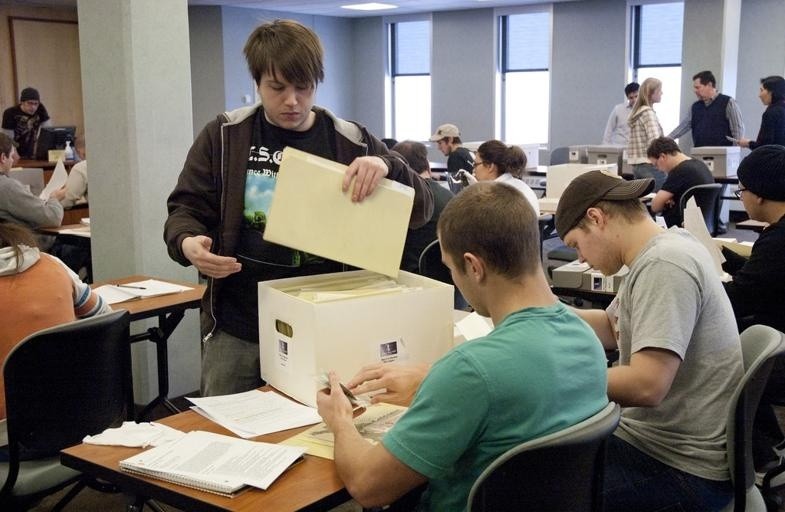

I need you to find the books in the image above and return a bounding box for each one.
[92,278,195,304]
[119,429,306,500]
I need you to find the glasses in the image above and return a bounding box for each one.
[472,160,484,167]
[733,187,748,198]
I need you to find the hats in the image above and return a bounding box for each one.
[428,124,459,142]
[554,170,656,240]
[19,87,40,102]
[736,144,785,203]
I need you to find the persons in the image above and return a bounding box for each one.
[391,138,456,275]
[472,138,543,220]
[318,177,612,512]
[1,210,116,464]
[722,144,785,487]
[554,167,746,512]
[161,14,435,400]
[428,123,478,189]
[0,84,50,161]
[597,69,785,233]
[0,131,93,284]
[57,132,89,209]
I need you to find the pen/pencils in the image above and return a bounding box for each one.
[320,375,357,401]
[117,284,147,290]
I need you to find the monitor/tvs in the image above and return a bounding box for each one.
[35,126,76,160]
